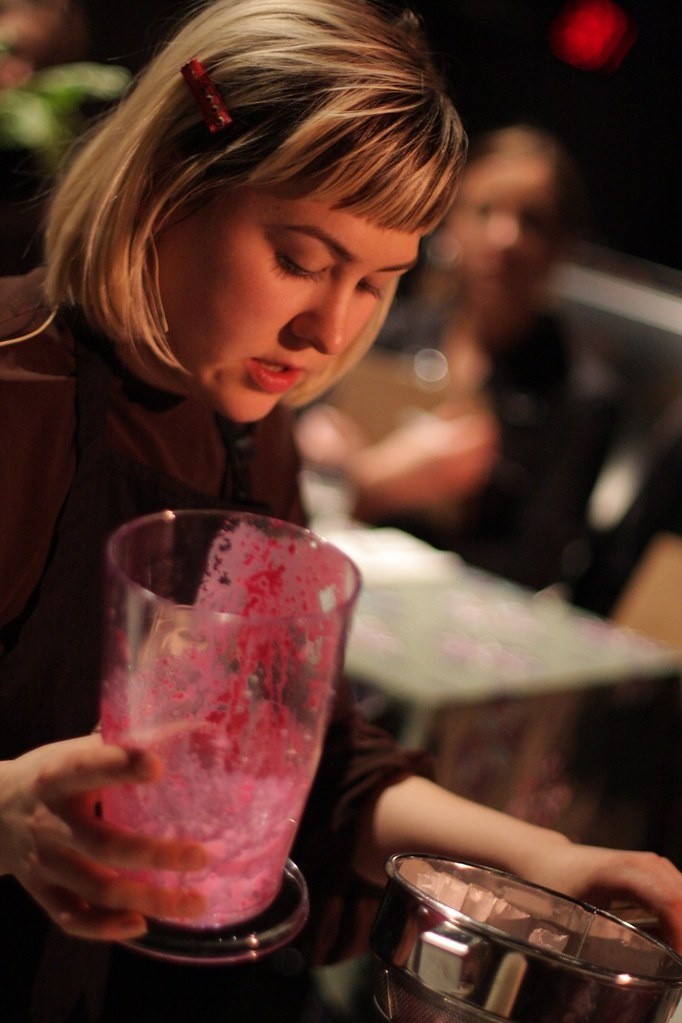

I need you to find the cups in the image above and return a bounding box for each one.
[96,510,361,961]
[370,853,682,1023]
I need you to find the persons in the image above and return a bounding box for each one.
[0,0,682,1023]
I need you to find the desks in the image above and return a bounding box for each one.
[304,513,682,960]
